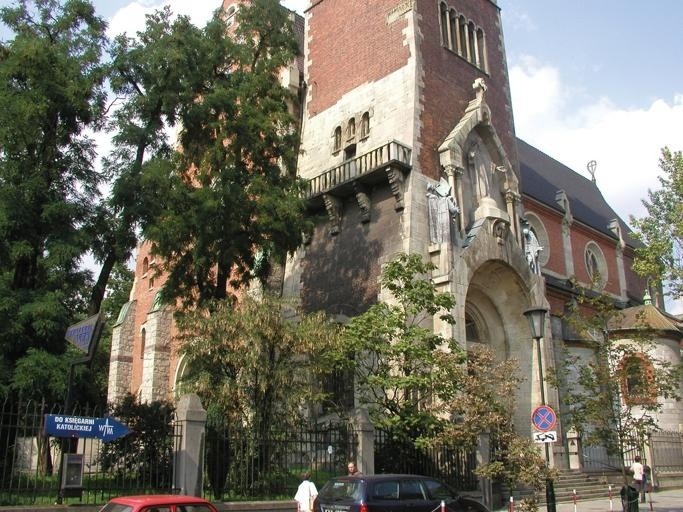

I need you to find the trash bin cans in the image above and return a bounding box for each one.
[620,486,639,512]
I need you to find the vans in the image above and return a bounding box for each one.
[309,471,492,512]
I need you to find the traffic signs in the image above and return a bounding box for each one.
[42,412,135,444]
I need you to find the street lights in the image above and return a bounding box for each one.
[520,304,557,512]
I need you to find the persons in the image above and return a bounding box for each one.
[347,461,363,499]
[294,472,319,512]
[629,456,645,491]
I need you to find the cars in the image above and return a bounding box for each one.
[96,493,220,512]
